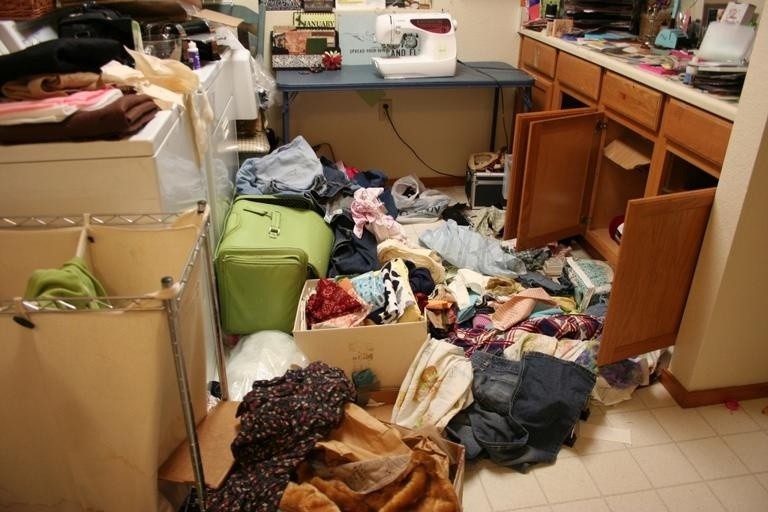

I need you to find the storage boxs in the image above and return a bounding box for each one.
[158,398,467,512]
[293,278,428,390]
[465,165,507,210]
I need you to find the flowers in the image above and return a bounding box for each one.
[322,50,343,70]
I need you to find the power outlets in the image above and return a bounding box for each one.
[380,100,392,119]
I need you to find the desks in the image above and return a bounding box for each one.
[276,62,535,152]
[504,30,741,368]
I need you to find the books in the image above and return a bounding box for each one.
[561,22,747,102]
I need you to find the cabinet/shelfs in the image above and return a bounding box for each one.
[1,49,239,251]
